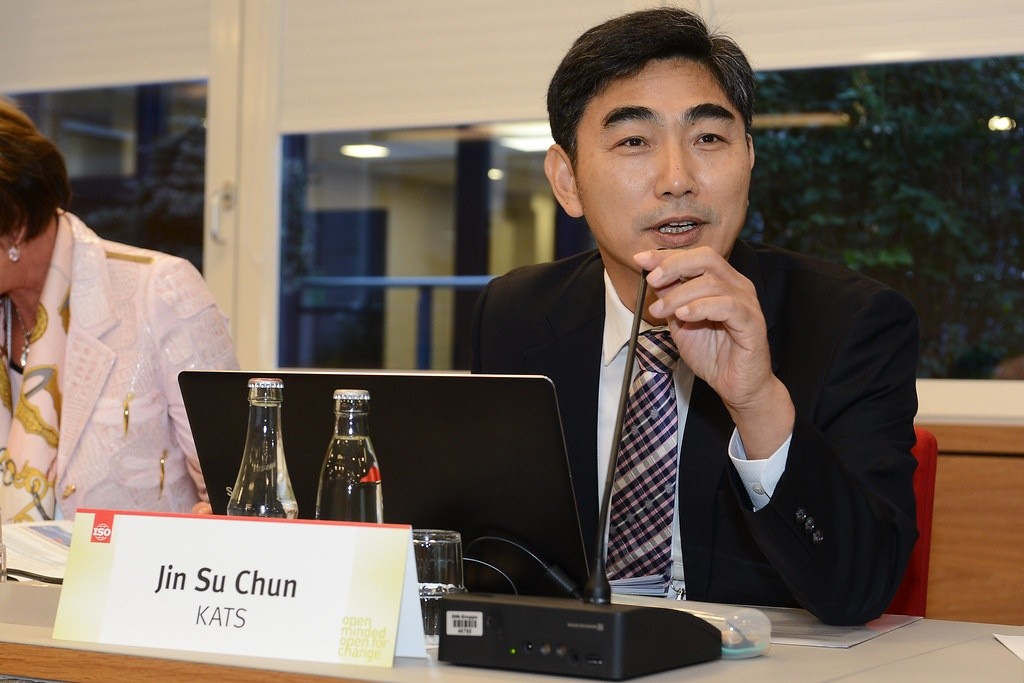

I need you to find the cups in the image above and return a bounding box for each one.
[414,529,463,647]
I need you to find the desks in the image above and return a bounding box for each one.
[0,591,1024,683]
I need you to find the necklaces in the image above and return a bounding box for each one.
[15,308,37,367]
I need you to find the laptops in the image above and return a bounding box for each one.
[179,371,592,637]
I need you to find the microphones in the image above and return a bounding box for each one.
[437,247,724,681]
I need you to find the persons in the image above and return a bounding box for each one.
[0,95,239,524]
[464,6,918,627]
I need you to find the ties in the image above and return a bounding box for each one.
[604,330,682,595]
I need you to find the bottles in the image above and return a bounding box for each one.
[315,389,382,523]
[228,377,299,520]
